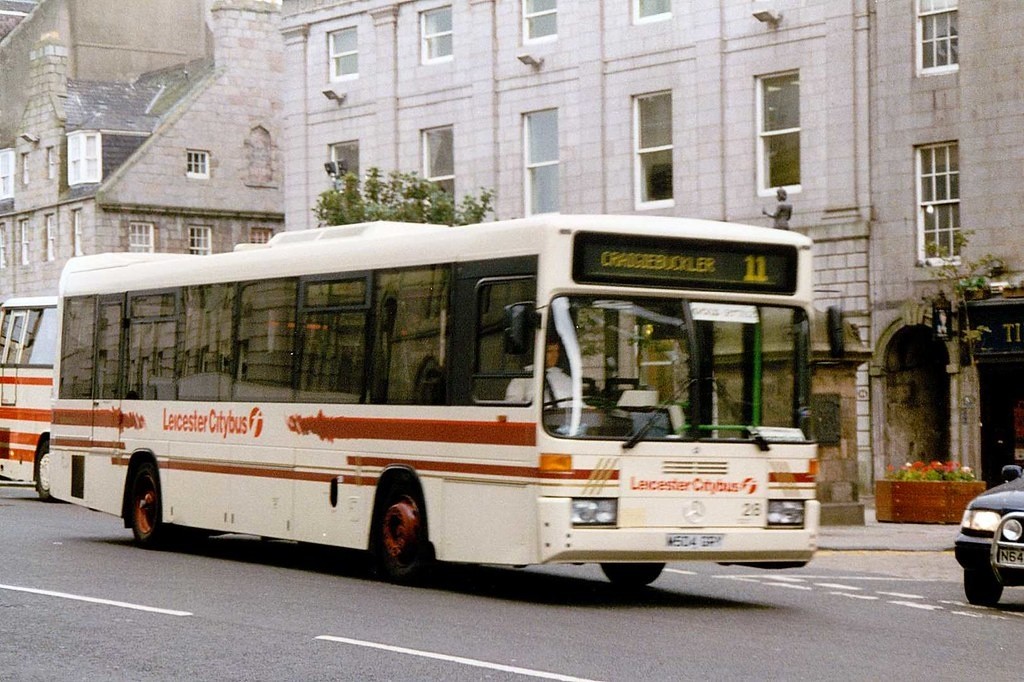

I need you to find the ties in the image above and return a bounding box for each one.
[544,374,558,408]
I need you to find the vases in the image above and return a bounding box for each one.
[876,479,986,524]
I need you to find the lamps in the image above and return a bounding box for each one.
[322,91,347,102]
[752,10,782,24]
[518,54,544,66]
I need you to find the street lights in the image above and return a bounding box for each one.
[323,159,348,225]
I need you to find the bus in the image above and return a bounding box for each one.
[0,295,58,502]
[48,212,848,590]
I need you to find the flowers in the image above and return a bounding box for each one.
[885,461,975,481]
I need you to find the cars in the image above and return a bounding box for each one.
[953,465,1024,605]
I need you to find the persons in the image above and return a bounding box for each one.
[503,337,595,411]
